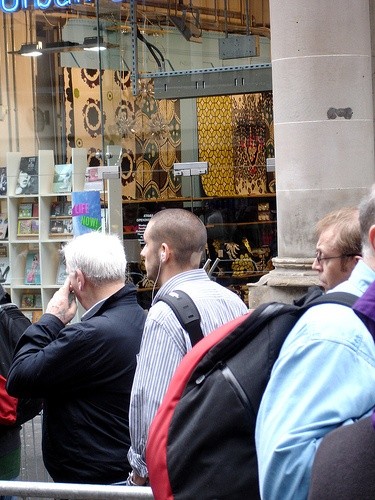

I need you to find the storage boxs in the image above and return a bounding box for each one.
[21,310,32,323]
[20,293,34,308]
[51,202,64,216]
[18,203,32,217]
[63,201,72,216]
[57,196,67,203]
[31,220,39,234]
[63,219,73,233]
[34,294,43,308]
[33,310,43,324]
[32,203,38,217]
[17,219,31,234]
[50,218,64,233]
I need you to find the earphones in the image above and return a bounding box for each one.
[160,251,166,259]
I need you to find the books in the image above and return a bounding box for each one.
[0,156,108,324]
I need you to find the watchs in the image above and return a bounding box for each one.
[127,475,136,486]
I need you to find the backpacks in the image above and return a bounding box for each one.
[144,284,361,500]
[0,291,44,428]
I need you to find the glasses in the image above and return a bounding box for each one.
[314,251,362,265]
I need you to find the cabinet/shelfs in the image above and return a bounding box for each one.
[0,147,123,324]
[122,193,277,310]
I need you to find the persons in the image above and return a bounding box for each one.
[253,186,375,500]
[200,198,252,240]
[5,232,147,500]
[16,159,38,194]
[0,283,21,500]
[126,208,247,485]
[311,210,363,292]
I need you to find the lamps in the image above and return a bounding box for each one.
[73,36,121,51]
[7,43,47,58]
